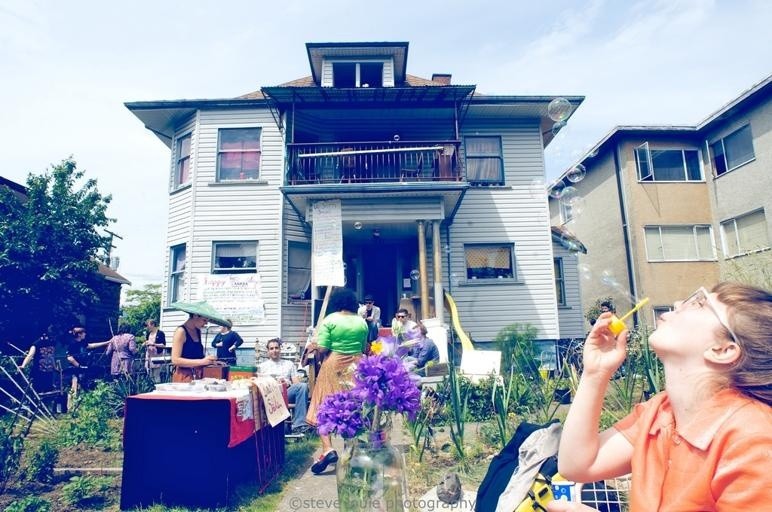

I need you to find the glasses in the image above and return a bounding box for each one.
[364,301,373,304]
[678,285,741,347]
[397,315,406,318]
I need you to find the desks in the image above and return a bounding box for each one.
[120,379,287,512]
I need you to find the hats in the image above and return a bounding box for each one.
[170,300,232,328]
[364,295,376,301]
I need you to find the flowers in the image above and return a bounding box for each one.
[315,341,423,511]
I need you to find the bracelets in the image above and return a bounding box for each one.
[78,363,82,368]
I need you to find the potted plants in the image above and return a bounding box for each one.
[643,385,649,401]
[555,380,570,404]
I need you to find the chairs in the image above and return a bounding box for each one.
[255,339,305,442]
[400,153,424,182]
[338,148,358,182]
[460,350,502,385]
[420,319,449,377]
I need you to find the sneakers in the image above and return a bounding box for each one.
[292,425,314,435]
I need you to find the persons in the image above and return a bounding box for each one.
[258,339,309,433]
[356,294,381,344]
[409,323,440,377]
[391,308,417,335]
[210,318,244,366]
[304,288,372,475]
[141,318,167,383]
[548,278,771,511]
[600,300,627,381]
[16,325,64,416]
[67,326,115,394]
[105,323,140,380]
[170,312,227,383]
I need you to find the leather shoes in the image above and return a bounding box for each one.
[312,450,338,473]
